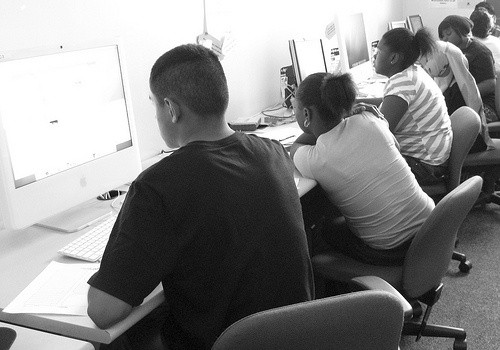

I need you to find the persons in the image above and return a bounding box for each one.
[290,72,436,265]
[438,15,497,123]
[470,9,500,120]
[87,44,315,350]
[418,40,500,153]
[475,2,500,36]
[372,28,453,186]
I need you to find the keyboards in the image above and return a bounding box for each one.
[58,213,119,262]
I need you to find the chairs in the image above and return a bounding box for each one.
[464,71,499,207]
[420,107,480,271]
[211,274,412,350]
[311,176,483,350]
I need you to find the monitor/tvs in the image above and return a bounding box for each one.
[0,13,424,235]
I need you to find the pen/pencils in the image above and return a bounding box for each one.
[279,135,295,141]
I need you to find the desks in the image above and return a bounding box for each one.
[0,76,385,350]
